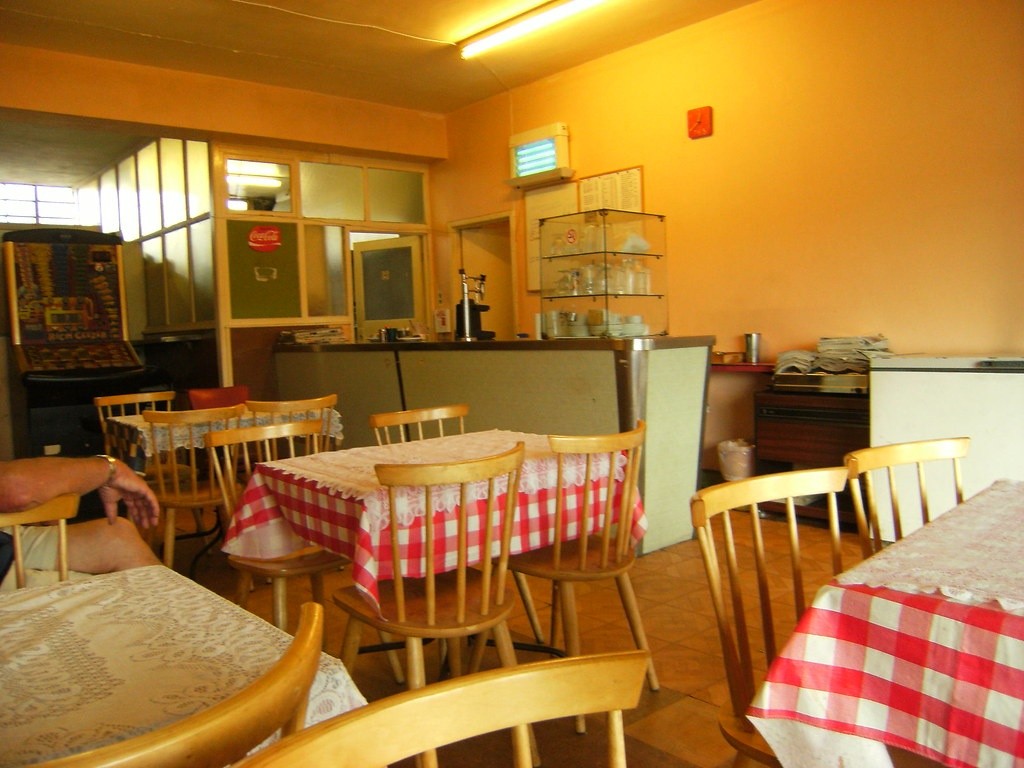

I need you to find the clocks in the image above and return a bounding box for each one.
[687,106,712,139]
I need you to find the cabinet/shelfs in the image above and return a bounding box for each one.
[539,208,669,339]
[751,392,871,527]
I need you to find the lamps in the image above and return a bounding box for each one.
[509,123,571,178]
[455,0,604,60]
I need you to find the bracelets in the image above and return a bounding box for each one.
[92,455,116,485]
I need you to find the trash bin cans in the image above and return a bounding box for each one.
[717,438,755,512]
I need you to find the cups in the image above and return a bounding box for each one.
[744,333,761,363]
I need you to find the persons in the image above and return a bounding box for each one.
[0,453,163,597]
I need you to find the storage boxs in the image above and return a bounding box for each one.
[711,352,743,364]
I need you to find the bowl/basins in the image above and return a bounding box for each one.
[543,308,645,338]
[556,261,652,296]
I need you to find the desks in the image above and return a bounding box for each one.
[711,362,774,372]
[220,428,649,681]
[0,564,371,768]
[749,477,1024,768]
[107,406,344,580]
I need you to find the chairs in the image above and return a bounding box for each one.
[0,390,976,768]
